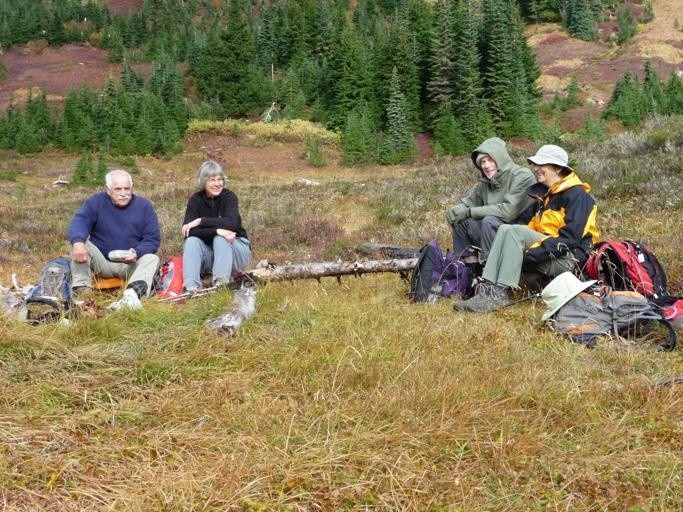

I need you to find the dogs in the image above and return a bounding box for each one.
[207,286,257,338]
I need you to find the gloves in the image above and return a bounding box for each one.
[445,203,471,225]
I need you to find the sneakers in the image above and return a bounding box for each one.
[124,287,143,309]
[454,281,509,312]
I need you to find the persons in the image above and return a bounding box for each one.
[445,137,537,263]
[454,145,601,314]
[68,169,161,310]
[182,161,253,298]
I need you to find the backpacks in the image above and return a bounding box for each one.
[588,239,683,321]
[410,238,468,302]
[555,290,676,351]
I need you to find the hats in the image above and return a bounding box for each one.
[527,144,574,172]
[539,270,598,321]
[476,152,489,166]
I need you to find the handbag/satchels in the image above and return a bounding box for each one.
[157,255,183,299]
